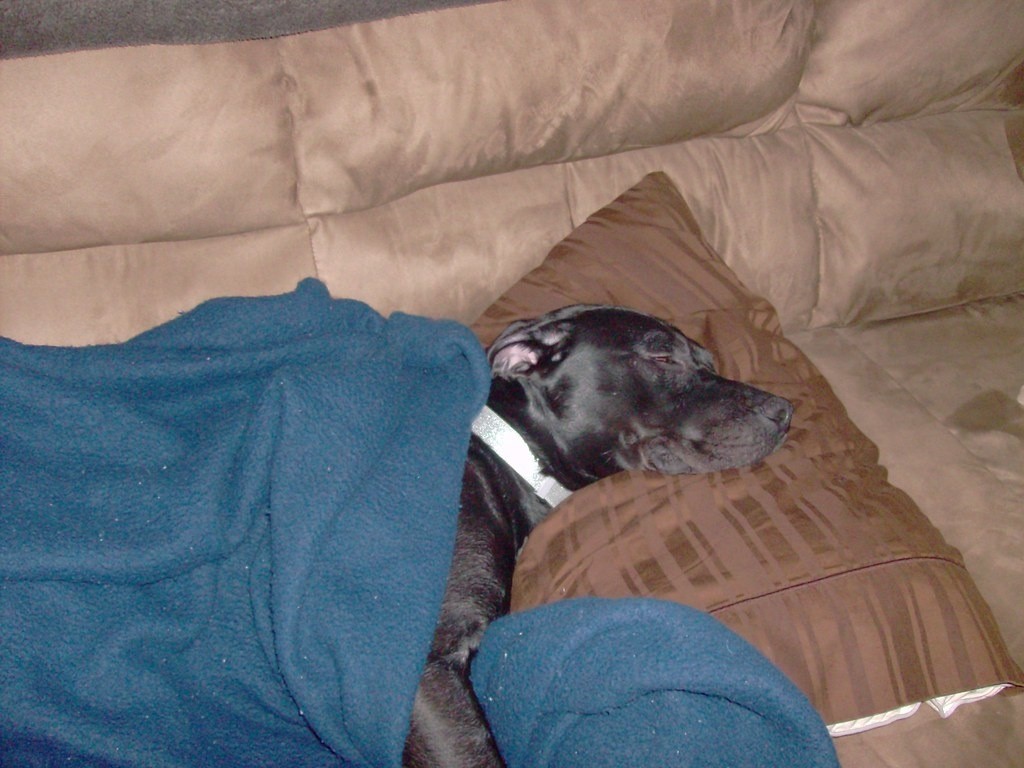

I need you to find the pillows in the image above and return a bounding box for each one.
[468,172,1024,736]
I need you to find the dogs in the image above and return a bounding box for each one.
[405,302,794,768]
